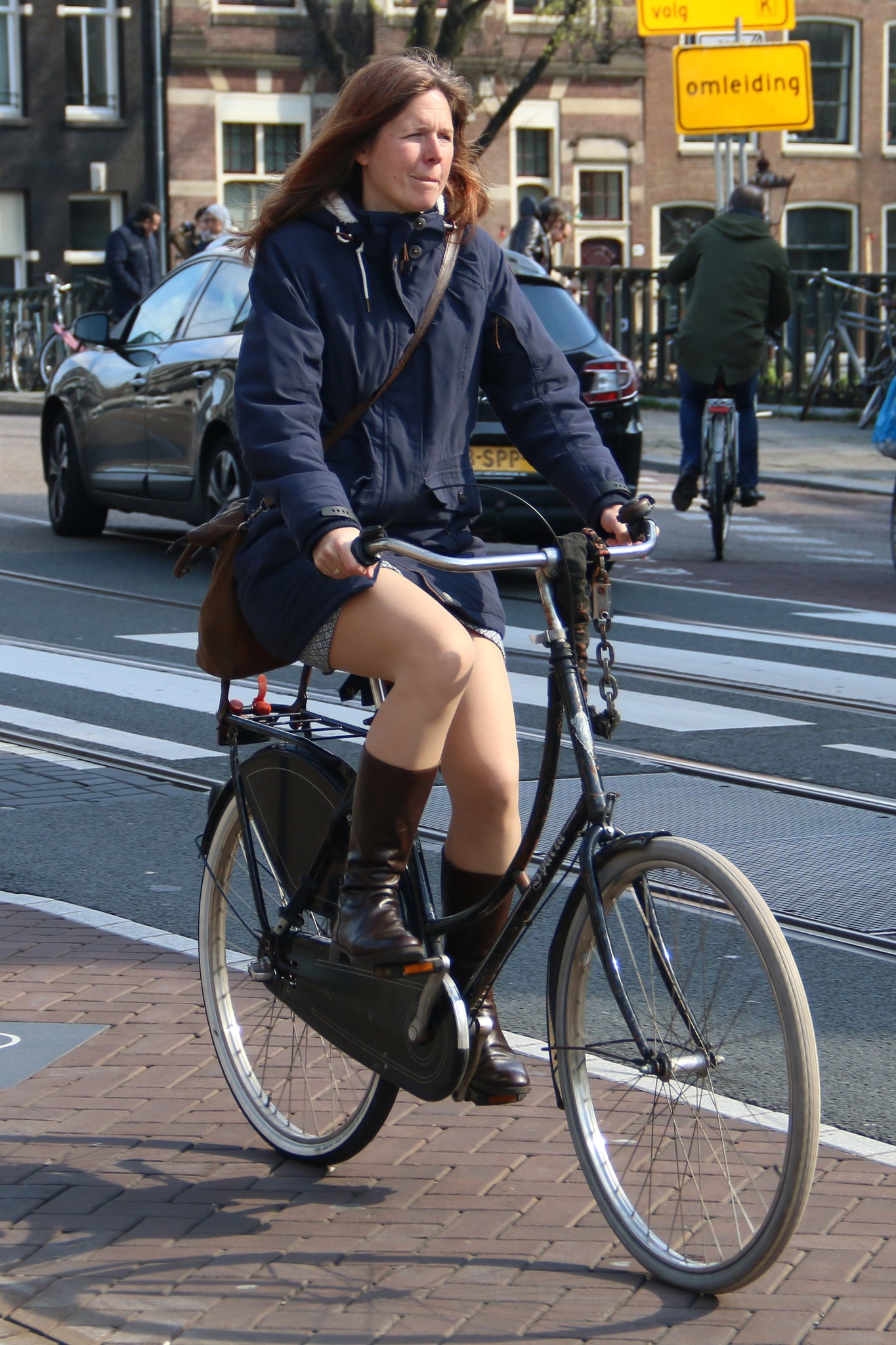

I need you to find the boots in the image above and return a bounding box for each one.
[324,743,441,963]
[438,846,533,1100]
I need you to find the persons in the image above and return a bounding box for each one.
[662,184,793,510]
[104,204,162,323]
[234,54,658,1118]
[204,203,238,240]
[506,195,582,296]
[169,208,210,258]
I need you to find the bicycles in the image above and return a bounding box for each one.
[649,323,795,560]
[799,268,896,429]
[8,282,80,392]
[194,493,820,1292]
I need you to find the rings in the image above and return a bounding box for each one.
[331,568,340,576]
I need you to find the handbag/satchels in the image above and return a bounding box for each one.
[170,495,301,680]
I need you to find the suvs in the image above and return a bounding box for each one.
[38,236,643,580]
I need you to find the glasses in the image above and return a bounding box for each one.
[201,217,217,223]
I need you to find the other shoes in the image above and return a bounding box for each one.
[672,479,696,511]
[741,488,765,506]
